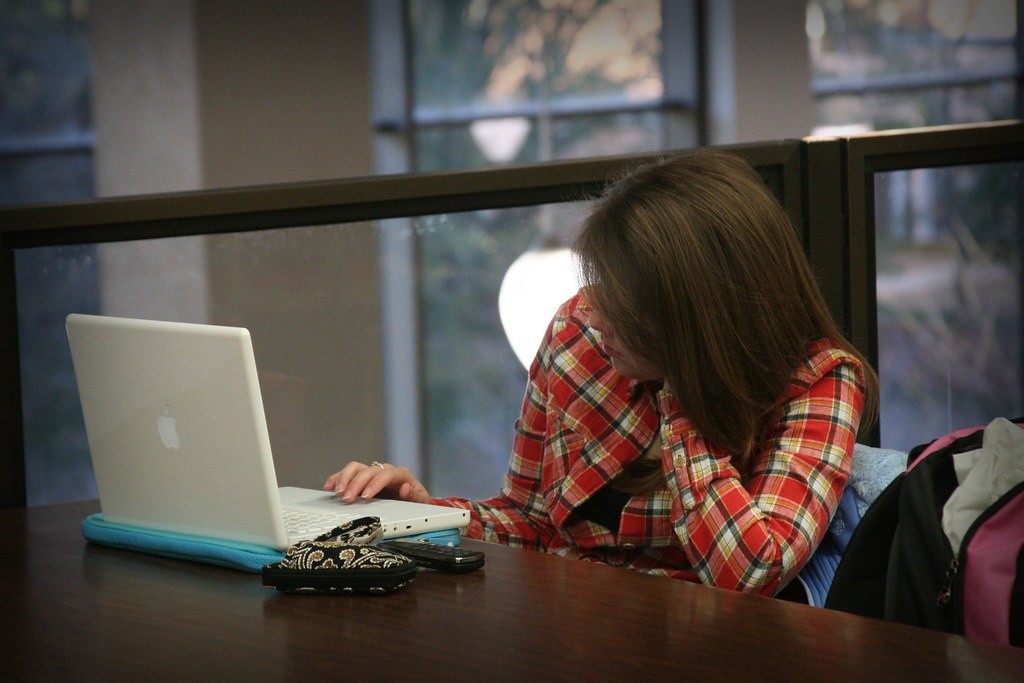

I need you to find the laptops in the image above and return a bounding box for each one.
[66,313,471,553]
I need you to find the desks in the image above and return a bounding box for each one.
[0,501,1024,682]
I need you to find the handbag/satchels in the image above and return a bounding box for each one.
[823,415,1024,645]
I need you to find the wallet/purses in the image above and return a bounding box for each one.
[261,517,418,596]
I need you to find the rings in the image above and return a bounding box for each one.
[370,461,385,469]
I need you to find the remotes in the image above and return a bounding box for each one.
[377,538,485,571]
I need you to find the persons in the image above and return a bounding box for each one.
[323,152,879,596]
